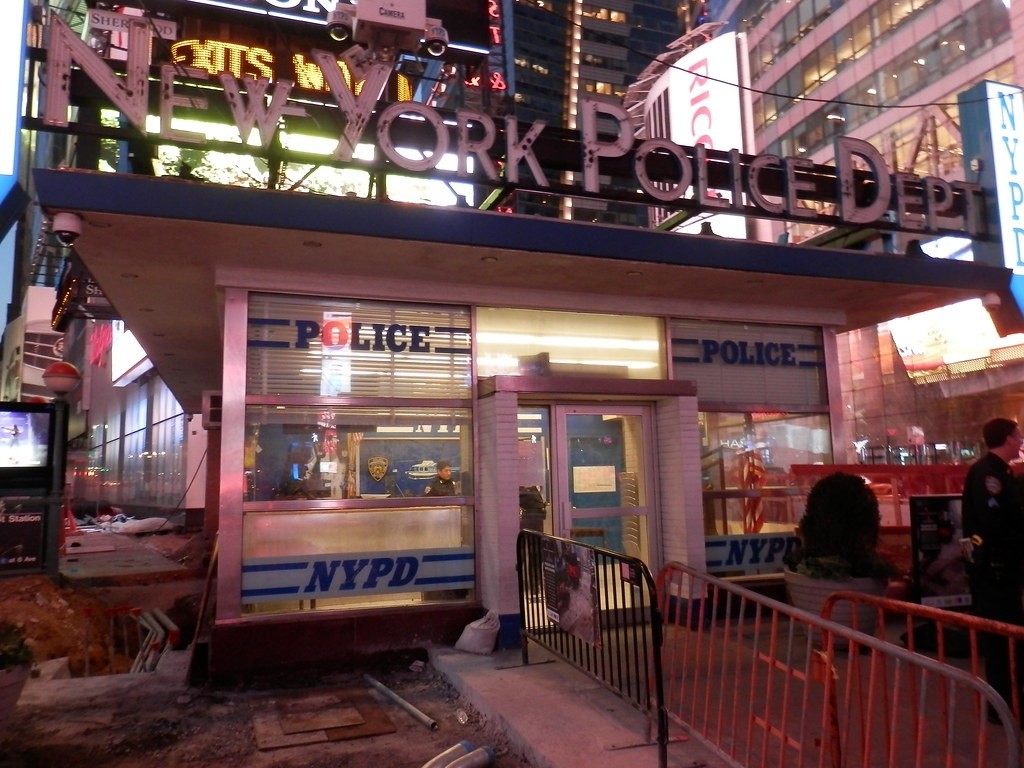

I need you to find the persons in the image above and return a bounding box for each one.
[960,418,1024,727]
[924,523,967,596]
[423,461,456,497]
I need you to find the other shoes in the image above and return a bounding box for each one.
[988,708,1024,729]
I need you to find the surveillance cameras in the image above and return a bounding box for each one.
[424,26,449,56]
[326,10,353,41]
[53,212,82,247]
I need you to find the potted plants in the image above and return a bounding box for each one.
[783,471,891,655]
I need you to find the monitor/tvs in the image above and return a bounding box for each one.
[0,401,56,479]
